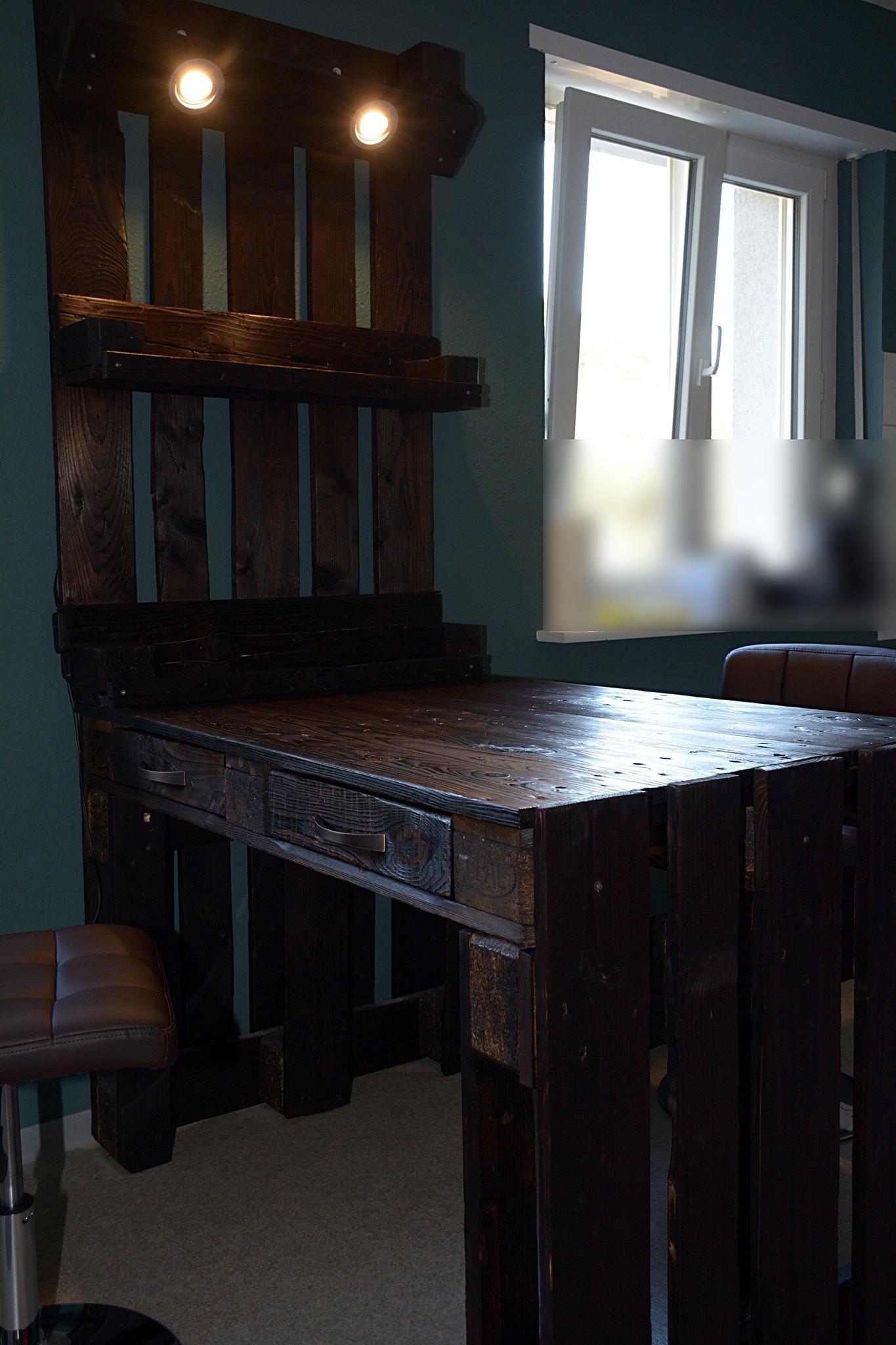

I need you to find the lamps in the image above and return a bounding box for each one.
[168,53,226,116]
[350,100,399,150]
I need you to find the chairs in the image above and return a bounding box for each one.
[719,642,896,719]
[0,923,180,1345]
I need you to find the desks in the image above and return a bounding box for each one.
[119,678,895,1345]
